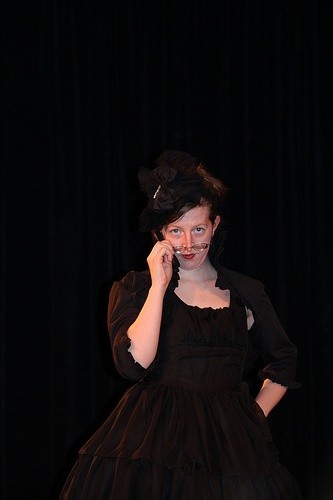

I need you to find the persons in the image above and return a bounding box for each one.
[58,167,301,500]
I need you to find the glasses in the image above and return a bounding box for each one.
[153,233,214,255]
